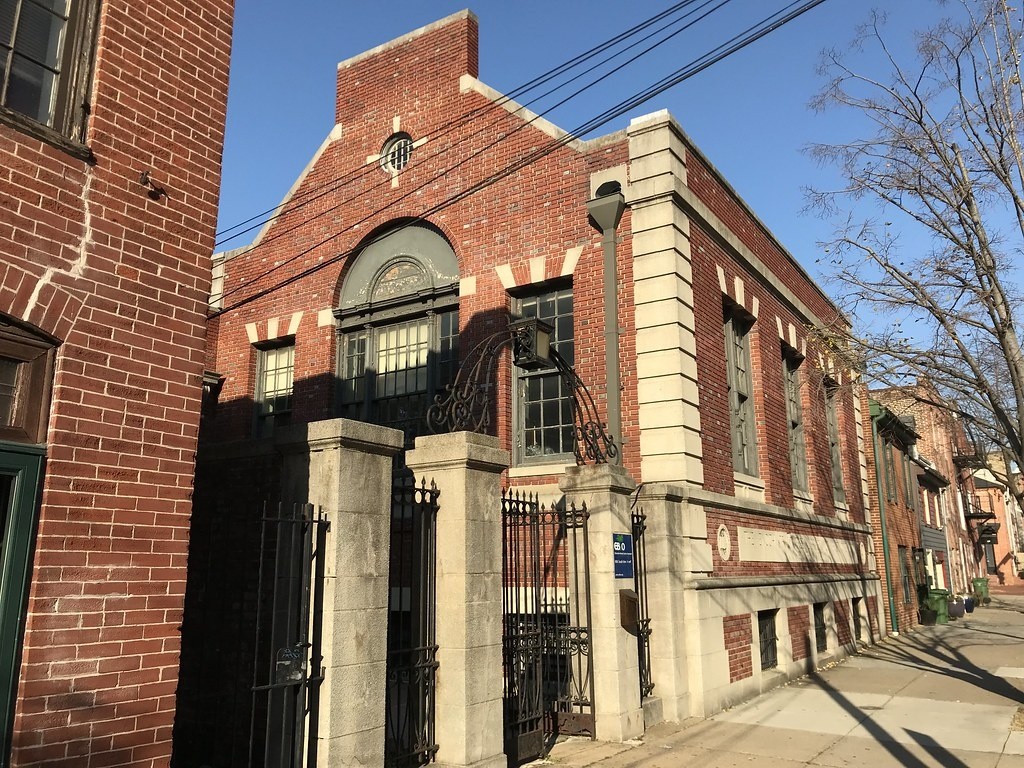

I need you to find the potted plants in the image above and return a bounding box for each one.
[919,600,939,626]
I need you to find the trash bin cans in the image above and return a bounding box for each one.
[973,578,990,597]
[927,589,949,624]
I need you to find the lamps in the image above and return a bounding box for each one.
[506,315,557,370]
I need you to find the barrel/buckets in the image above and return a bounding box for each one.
[966,599,974,613]
[919,609,939,626]
